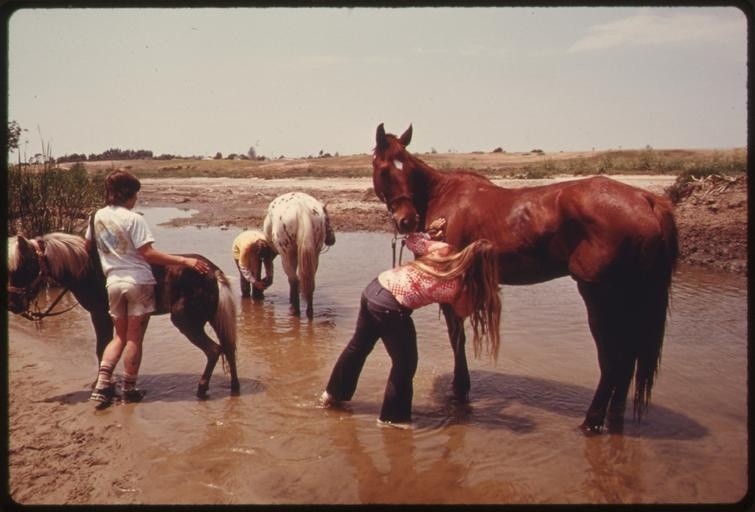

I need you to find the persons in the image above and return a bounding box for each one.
[229,228,274,302]
[82,167,207,408]
[316,218,507,432]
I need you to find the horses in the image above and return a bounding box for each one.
[6,231,241,400]
[262,190,336,320]
[371,122,679,438]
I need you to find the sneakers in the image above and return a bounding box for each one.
[318,391,340,409]
[377,420,419,430]
[87,388,109,407]
[121,387,146,405]
